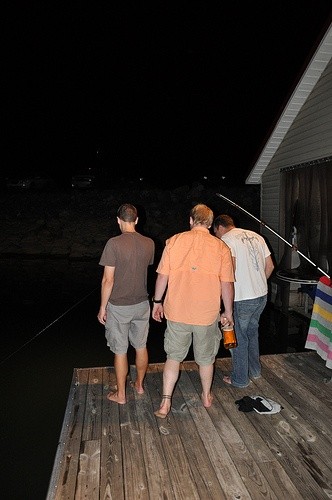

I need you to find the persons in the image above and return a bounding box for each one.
[212,213,275,388]
[97,204,155,404]
[152,204,236,418]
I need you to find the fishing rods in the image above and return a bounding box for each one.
[0,284,101,366]
[214,191,331,279]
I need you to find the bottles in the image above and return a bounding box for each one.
[221,318,237,349]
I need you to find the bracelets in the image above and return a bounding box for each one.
[151,296,162,303]
